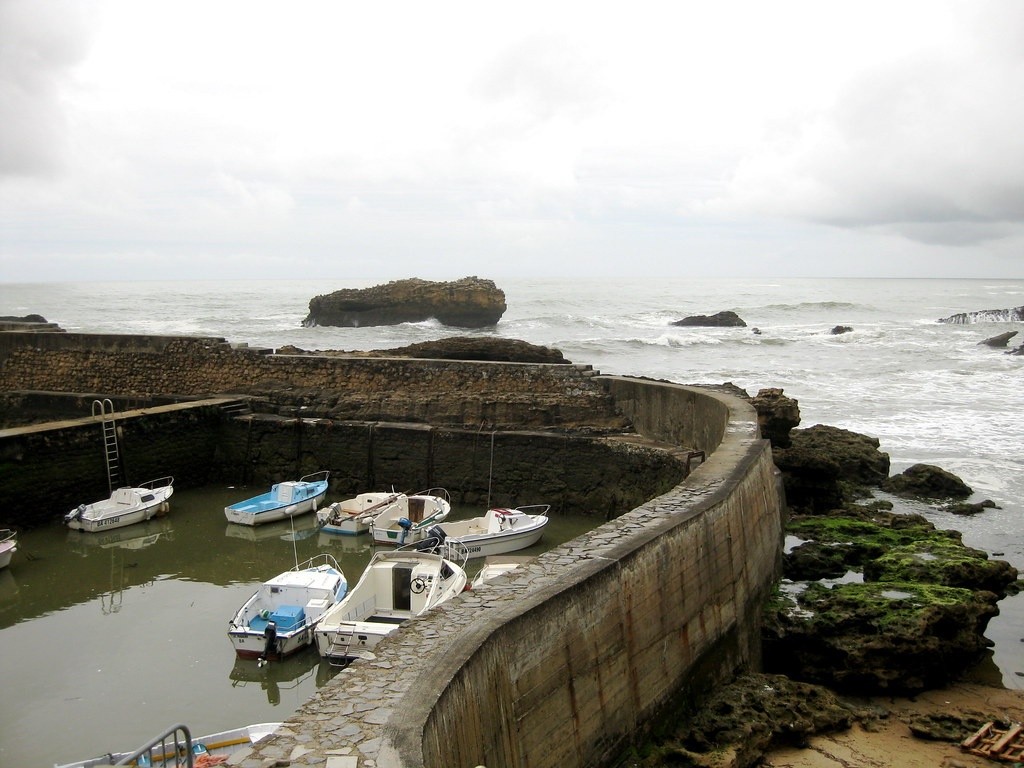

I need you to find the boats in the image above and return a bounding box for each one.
[62,476,176,533]
[224,470,332,527]
[0,528,20,571]
[314,533,372,563]
[227,514,348,665]
[420,504,550,560]
[225,512,321,541]
[470,559,524,591]
[368,488,452,547]
[228,644,320,707]
[316,493,406,534]
[52,722,285,768]
[63,516,175,560]
[0,567,21,613]
[313,534,469,667]
